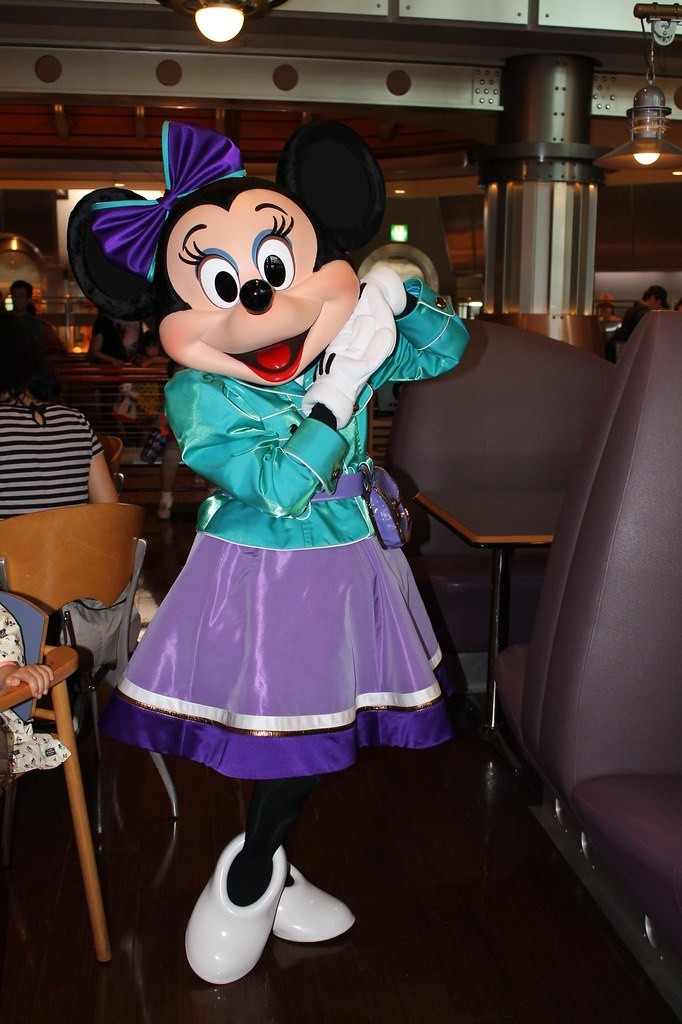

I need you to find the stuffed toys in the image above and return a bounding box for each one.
[65,118,467,986]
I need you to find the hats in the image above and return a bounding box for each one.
[649,285,672,310]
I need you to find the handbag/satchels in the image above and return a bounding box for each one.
[140,428,169,465]
[48,580,141,679]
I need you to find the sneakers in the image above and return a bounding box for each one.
[158,491,173,520]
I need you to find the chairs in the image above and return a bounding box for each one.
[0,591,110,962]
[0,503,181,817]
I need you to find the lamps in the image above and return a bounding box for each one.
[593,18,682,171]
[156,0,288,43]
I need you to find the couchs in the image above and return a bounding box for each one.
[493,310,682,1022]
[383,317,616,652]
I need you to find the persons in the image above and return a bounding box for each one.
[1,279,161,368]
[0,601,54,777]
[605,284,682,364]
[0,315,120,521]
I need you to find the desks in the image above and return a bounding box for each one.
[413,489,565,777]
